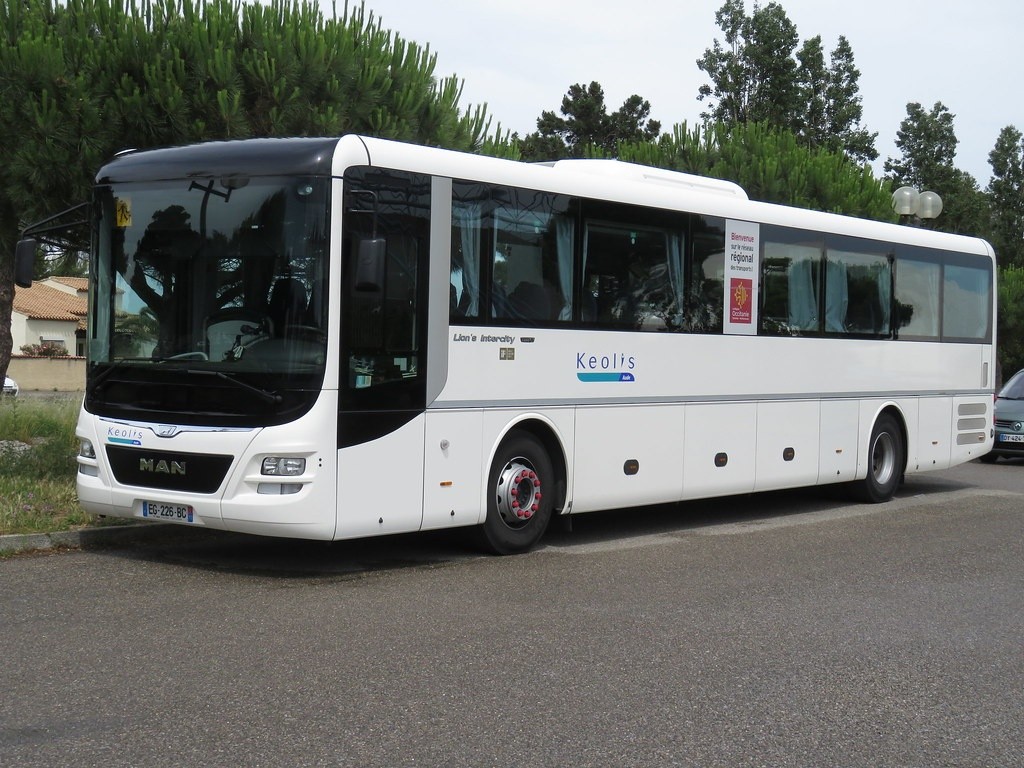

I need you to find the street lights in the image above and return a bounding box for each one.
[183,171,252,360]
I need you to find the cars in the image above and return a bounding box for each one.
[2,374,20,399]
[975,367,1024,467]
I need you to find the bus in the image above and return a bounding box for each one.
[12,133,1001,561]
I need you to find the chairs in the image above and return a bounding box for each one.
[200,307,274,362]
[342,283,875,380]
